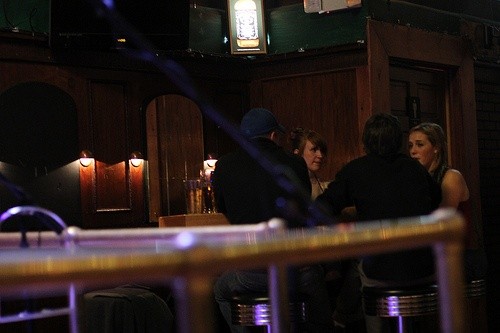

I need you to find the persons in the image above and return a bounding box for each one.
[289,124,330,201]
[211,108,312,333]
[312,112,441,333]
[407,121,470,280]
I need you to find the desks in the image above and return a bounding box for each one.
[159,213,229,228]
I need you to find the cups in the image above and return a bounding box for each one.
[201,182,216,214]
[184,180,201,215]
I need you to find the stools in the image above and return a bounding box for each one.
[233,295,304,333]
[376,277,485,333]
